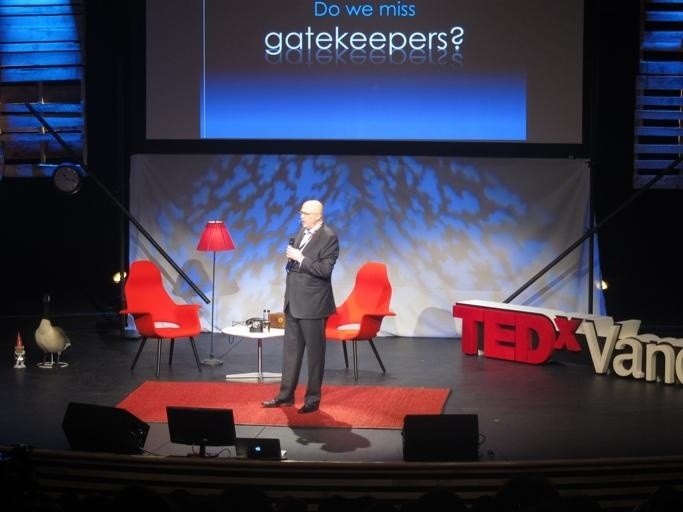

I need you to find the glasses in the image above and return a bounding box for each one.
[298,210,321,215]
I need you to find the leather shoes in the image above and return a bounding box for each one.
[299,402,321,414]
[260,395,296,407]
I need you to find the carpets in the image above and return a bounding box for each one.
[116,380,452,430]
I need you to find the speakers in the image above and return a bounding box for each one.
[401,413,478,460]
[61,401,149,455]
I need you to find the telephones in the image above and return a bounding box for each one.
[231,318,267,332]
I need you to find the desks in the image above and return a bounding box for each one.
[222,323,285,383]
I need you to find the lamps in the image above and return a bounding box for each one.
[196,220,236,368]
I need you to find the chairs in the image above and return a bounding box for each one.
[325,262,397,380]
[119,260,203,378]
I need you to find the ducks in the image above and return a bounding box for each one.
[34,294,72,367]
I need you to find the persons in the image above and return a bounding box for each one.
[262,200,339,414]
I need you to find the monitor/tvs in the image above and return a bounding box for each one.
[165,406,236,446]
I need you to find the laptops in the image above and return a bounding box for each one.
[236,438,286,458]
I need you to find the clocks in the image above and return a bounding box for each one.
[52,163,83,194]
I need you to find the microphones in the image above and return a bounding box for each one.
[287,238,294,262]
